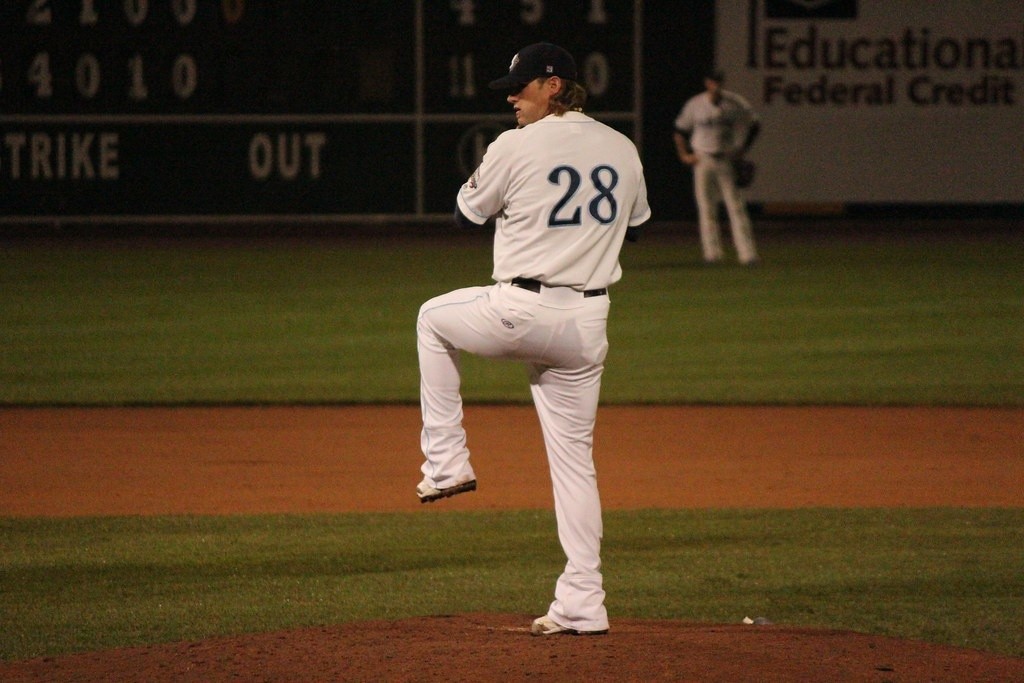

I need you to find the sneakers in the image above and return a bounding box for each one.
[532,615,608,635]
[417,477,476,503]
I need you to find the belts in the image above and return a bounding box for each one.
[511,277,607,299]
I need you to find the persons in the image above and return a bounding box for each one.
[673,71,763,264]
[416,42,652,635]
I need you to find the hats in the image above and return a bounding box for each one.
[489,41,577,90]
